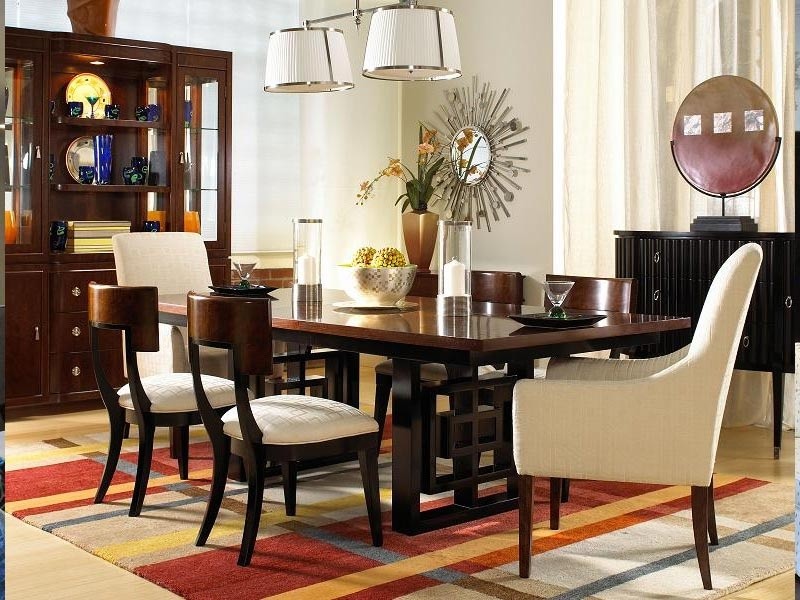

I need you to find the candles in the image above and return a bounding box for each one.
[444,257,465,297]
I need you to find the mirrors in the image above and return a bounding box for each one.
[415,76,530,230]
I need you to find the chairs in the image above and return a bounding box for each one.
[512,244,764,591]
[87,231,638,569]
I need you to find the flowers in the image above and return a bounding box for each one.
[353,121,486,213]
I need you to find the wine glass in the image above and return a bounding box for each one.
[85,96,100,118]
[233,258,261,289]
[542,281,575,318]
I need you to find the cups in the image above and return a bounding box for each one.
[148,210,166,232]
[93,135,113,185]
[49,220,67,251]
[185,100,192,128]
[135,104,160,122]
[68,102,83,118]
[105,104,120,120]
[123,167,138,185]
[5,210,16,243]
[184,212,202,233]
[50,155,54,181]
[143,221,160,232]
[79,166,94,184]
[131,157,148,185]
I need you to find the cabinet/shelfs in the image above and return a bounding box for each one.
[613,230,795,461]
[0,25,234,423]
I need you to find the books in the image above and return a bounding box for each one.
[65,220,132,254]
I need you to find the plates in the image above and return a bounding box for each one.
[508,314,607,328]
[208,285,278,294]
[66,136,95,184]
[333,301,418,308]
[65,73,113,121]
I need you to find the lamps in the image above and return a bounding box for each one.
[264,0,462,94]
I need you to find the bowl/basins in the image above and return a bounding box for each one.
[339,266,418,307]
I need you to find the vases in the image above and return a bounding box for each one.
[93,135,113,185]
[184,211,201,237]
[5,211,16,244]
[401,203,439,273]
[147,211,166,232]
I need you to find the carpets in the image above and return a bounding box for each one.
[5,402,794,600]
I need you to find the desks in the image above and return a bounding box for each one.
[157,288,691,535]
